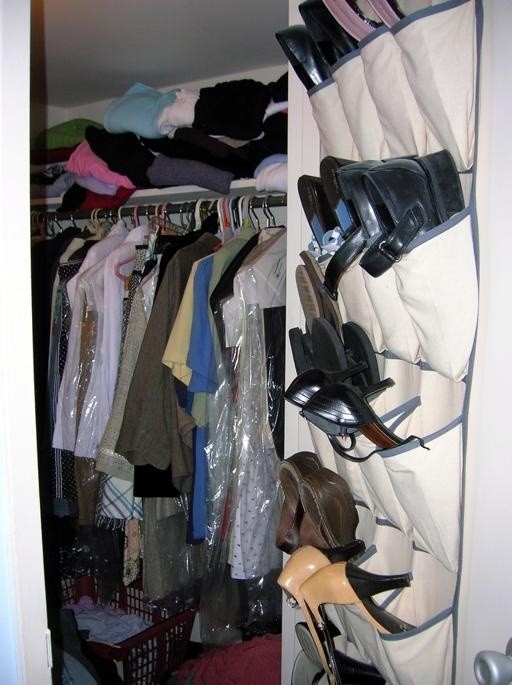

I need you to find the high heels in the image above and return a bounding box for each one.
[277,540,418,685]
[283,362,431,463]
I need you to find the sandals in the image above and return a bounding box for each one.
[289,250,381,388]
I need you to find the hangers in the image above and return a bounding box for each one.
[31,195,287,299]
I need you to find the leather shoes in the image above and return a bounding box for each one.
[276,0,404,93]
[297,150,466,301]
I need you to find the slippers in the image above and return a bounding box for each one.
[275,451,365,565]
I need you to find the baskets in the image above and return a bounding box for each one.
[46,566,196,685]
